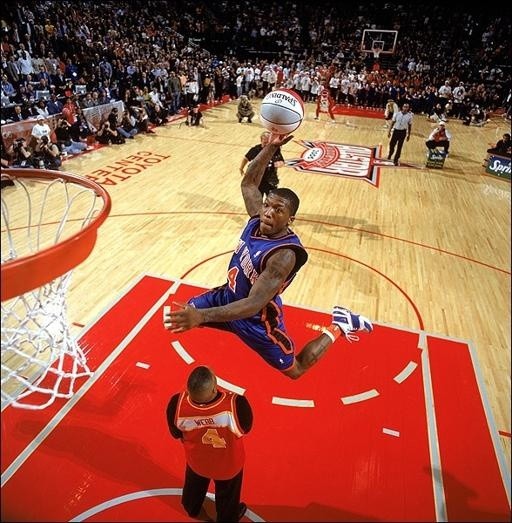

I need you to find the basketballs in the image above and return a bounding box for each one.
[260,87,307,136]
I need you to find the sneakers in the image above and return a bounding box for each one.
[239,503,247,521]
[332,306,374,345]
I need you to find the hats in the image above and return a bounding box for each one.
[38,115,45,121]
[439,122,446,127]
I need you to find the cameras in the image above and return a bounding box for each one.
[105,125,108,128]
[124,110,128,115]
[13,143,23,150]
[62,121,67,125]
[139,110,143,113]
[43,139,48,145]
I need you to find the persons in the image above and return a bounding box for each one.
[196,2,512,129]
[496,133,511,154]
[386,103,413,166]
[164,131,375,381]
[425,121,451,157]
[239,134,285,200]
[165,365,254,523]
[0,2,202,188]
